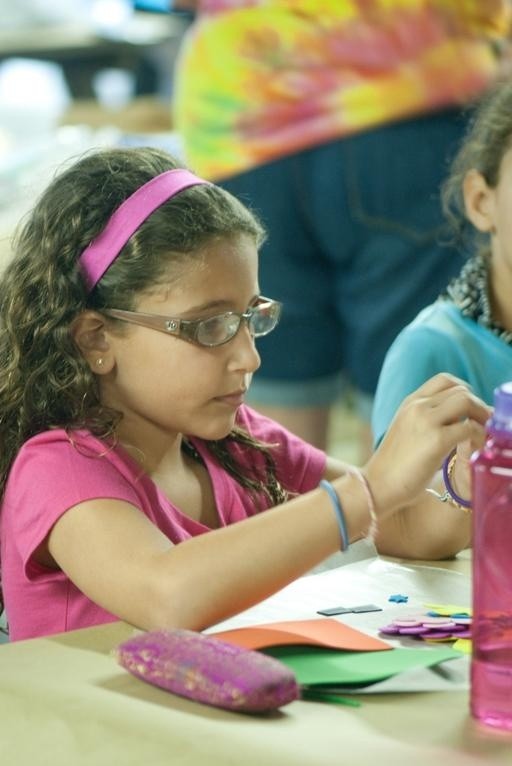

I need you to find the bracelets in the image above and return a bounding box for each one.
[319,477,349,552]
[446,452,472,516]
[343,462,378,544]
[441,447,472,507]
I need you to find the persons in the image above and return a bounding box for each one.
[1,145,495,647]
[163,0,511,465]
[372,72,512,456]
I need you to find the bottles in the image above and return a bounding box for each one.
[469,382,512,732]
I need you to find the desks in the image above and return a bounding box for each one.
[0,543,512,765]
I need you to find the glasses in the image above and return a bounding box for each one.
[101,295,282,348]
